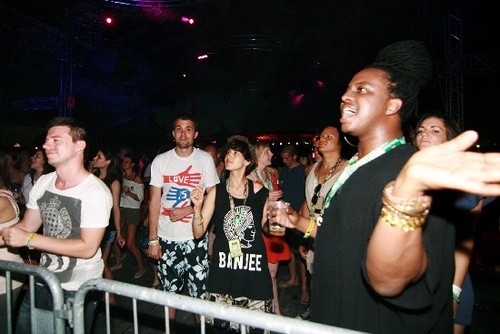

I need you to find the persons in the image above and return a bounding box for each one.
[0,64,500,334]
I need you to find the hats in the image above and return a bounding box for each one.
[227,135,258,176]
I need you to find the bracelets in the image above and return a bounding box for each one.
[148,243,158,245]
[193,214,204,225]
[303,219,315,237]
[186,206,191,215]
[451,294,460,302]
[26,232,37,250]
[147,237,158,240]
[149,240,158,243]
[452,284,462,296]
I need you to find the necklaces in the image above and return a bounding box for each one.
[324,158,341,181]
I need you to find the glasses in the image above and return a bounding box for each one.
[311,184,321,205]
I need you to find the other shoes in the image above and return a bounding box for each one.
[109,264,122,271]
[134,268,145,278]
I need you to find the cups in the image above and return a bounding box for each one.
[12,186,22,200]
[266,201,290,236]
